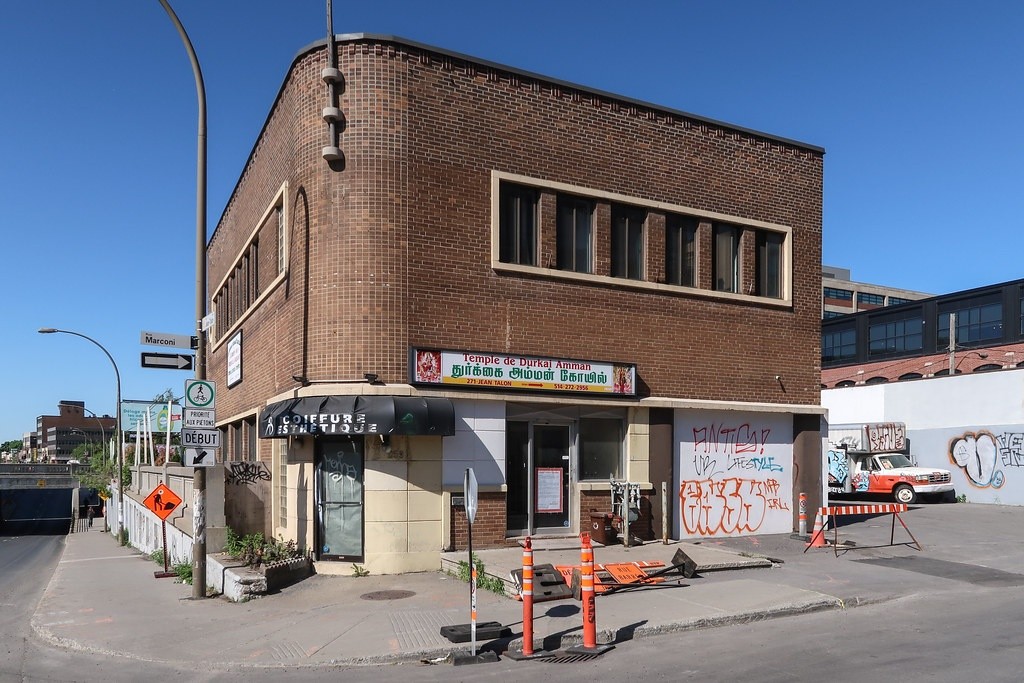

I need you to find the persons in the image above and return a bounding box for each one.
[87,506,95,528]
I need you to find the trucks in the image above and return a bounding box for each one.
[829,422,955,505]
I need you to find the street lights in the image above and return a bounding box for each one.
[68,425,94,459]
[38,327,127,548]
[57,403,109,533]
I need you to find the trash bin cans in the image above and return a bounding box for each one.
[588,508,617,545]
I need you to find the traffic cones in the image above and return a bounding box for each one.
[805,511,830,548]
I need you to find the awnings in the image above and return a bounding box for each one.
[258,395,455,438]
